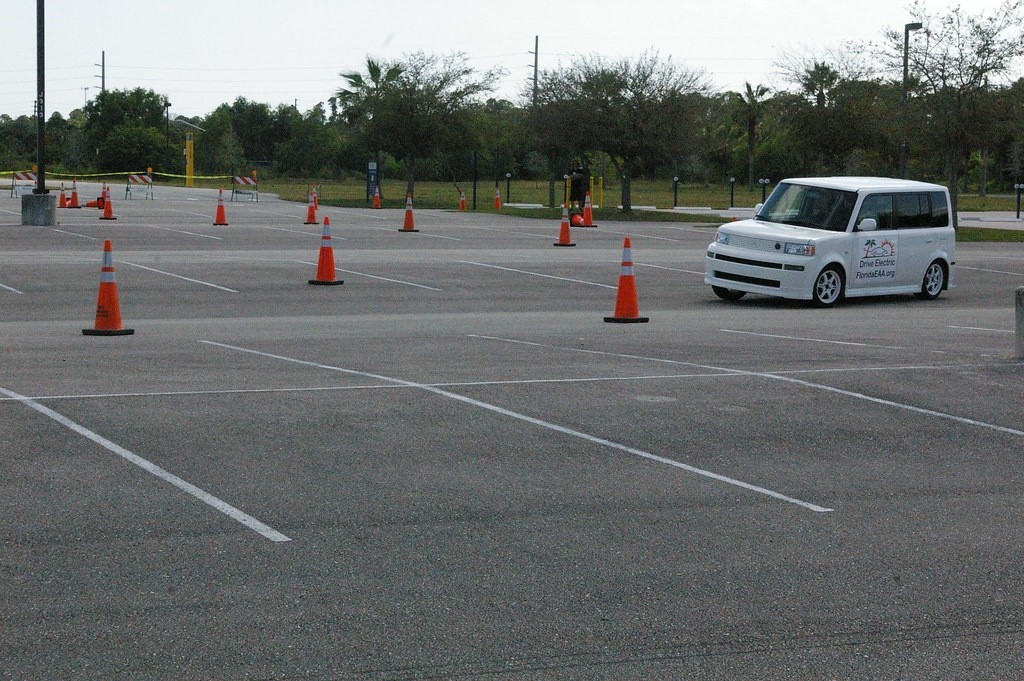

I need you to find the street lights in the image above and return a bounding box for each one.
[163,102,172,181]
[900,22,924,178]
[81,86,89,119]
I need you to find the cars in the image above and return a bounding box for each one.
[702,174,958,309]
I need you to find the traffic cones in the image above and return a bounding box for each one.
[569,211,586,227]
[370,185,383,209]
[55,182,68,208]
[67,177,82,209]
[577,191,600,228]
[307,216,345,286]
[552,202,577,247]
[311,184,320,210]
[212,187,229,227]
[100,179,107,209]
[601,236,651,324]
[302,190,320,225]
[99,187,117,220]
[458,187,468,212]
[490,187,503,210]
[65,197,72,207]
[82,239,136,336]
[398,191,420,232]
[82,195,104,209]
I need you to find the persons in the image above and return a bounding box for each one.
[569,160,586,211]
